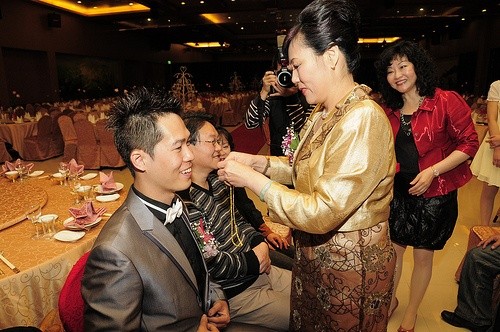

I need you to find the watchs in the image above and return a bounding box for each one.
[431,165,440,177]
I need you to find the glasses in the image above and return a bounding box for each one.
[188,137,223,146]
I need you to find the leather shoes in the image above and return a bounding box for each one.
[441,309,500,332]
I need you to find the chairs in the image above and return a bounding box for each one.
[57,249,93,332]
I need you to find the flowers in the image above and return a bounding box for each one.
[189,215,219,259]
[281,121,302,168]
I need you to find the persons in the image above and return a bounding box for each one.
[177,111,297,332]
[369,37,480,331]
[215,1,396,332]
[439,232,499,332]
[467,79,500,229]
[79,88,232,332]
[210,128,297,270]
[244,49,314,158]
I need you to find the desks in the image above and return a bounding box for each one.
[362,84,492,127]
[0,90,264,170]
[0,159,133,285]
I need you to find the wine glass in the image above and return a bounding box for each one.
[474,113,488,122]
[24,200,44,239]
[59,165,95,204]
[15,159,28,183]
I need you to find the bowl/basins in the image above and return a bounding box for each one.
[6,170,19,180]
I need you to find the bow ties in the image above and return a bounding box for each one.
[136,195,183,225]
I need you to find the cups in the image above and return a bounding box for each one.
[41,216,57,239]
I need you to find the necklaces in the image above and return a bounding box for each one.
[397,95,423,127]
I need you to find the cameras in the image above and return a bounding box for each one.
[273,48,295,87]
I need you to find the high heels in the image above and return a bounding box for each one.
[387,297,417,332]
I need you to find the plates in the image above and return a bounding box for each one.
[54,230,86,242]
[96,194,120,202]
[30,170,44,177]
[80,172,98,181]
[94,182,124,194]
[53,173,69,178]
[63,216,103,230]
[38,214,58,222]
